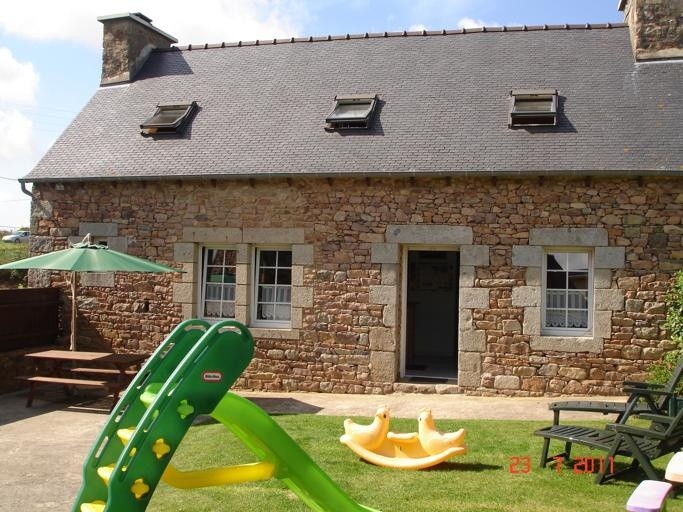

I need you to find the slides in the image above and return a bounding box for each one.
[141,382,382,512]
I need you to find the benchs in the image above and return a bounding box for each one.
[12,375,128,413]
[41,367,138,395]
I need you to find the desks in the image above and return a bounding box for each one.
[23,348,151,407]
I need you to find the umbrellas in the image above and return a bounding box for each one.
[0,232,188,351]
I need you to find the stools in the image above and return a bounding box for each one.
[624,480,673,512]
[664,451,683,484]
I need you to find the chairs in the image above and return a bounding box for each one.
[549,364,683,424]
[533,407,683,486]
